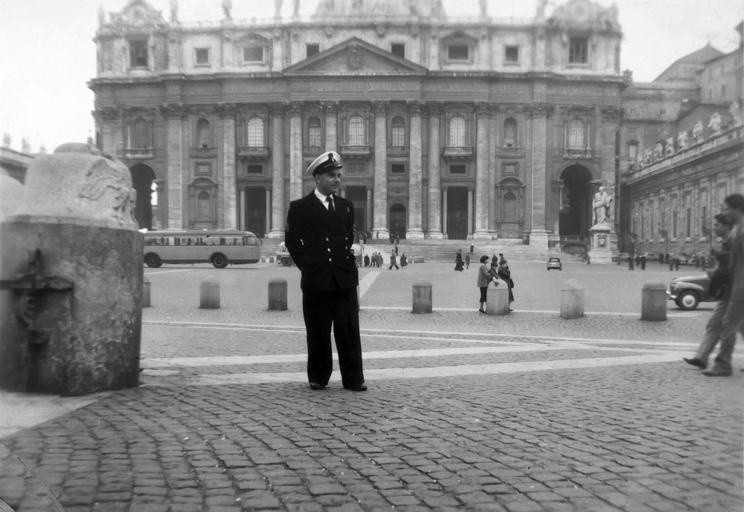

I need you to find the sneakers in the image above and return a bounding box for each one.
[510,308,513,311]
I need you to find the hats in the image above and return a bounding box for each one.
[305,151,343,175]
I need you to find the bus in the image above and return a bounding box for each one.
[139,229,263,269]
[273,237,363,268]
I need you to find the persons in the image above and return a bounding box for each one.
[351,226,407,269]
[456,242,515,314]
[628,249,710,271]
[683,213,744,369]
[285,151,368,391]
[703,194,744,375]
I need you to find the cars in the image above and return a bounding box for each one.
[667,269,715,313]
[616,250,711,268]
[546,256,562,271]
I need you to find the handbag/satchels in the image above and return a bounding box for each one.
[510,279,514,288]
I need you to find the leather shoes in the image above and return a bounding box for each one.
[682,356,706,367]
[309,382,325,390]
[701,361,732,376]
[343,382,367,392]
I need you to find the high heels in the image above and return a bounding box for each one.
[479,308,486,314]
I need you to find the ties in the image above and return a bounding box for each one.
[326,196,335,219]
